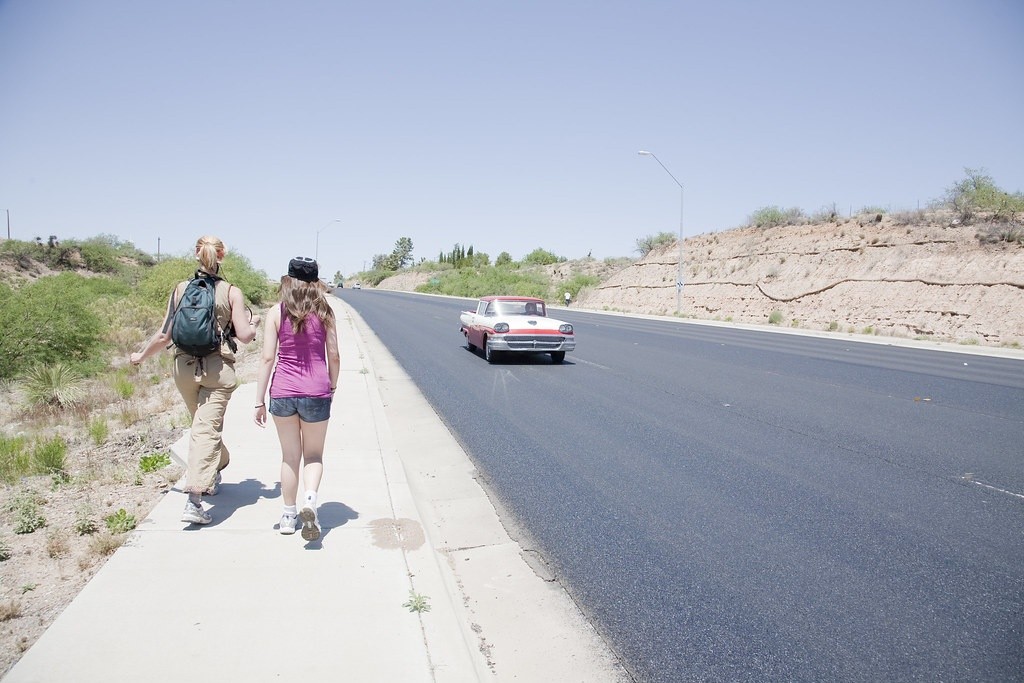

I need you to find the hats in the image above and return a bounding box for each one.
[288,257,318,278]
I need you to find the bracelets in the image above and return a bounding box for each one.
[255,403,265,408]
[331,386,337,390]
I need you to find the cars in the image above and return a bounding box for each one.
[352,283,361,289]
[337,283,343,288]
[461,295,576,362]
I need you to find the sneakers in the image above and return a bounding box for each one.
[279,514,297,534]
[300,504,321,542]
[181,500,212,524]
[205,471,221,495]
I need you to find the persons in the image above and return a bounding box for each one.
[130,235,261,524]
[252,257,340,540]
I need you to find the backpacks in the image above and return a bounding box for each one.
[170,268,233,357]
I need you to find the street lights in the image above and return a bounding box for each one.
[316,219,340,262]
[638,150,683,309]
